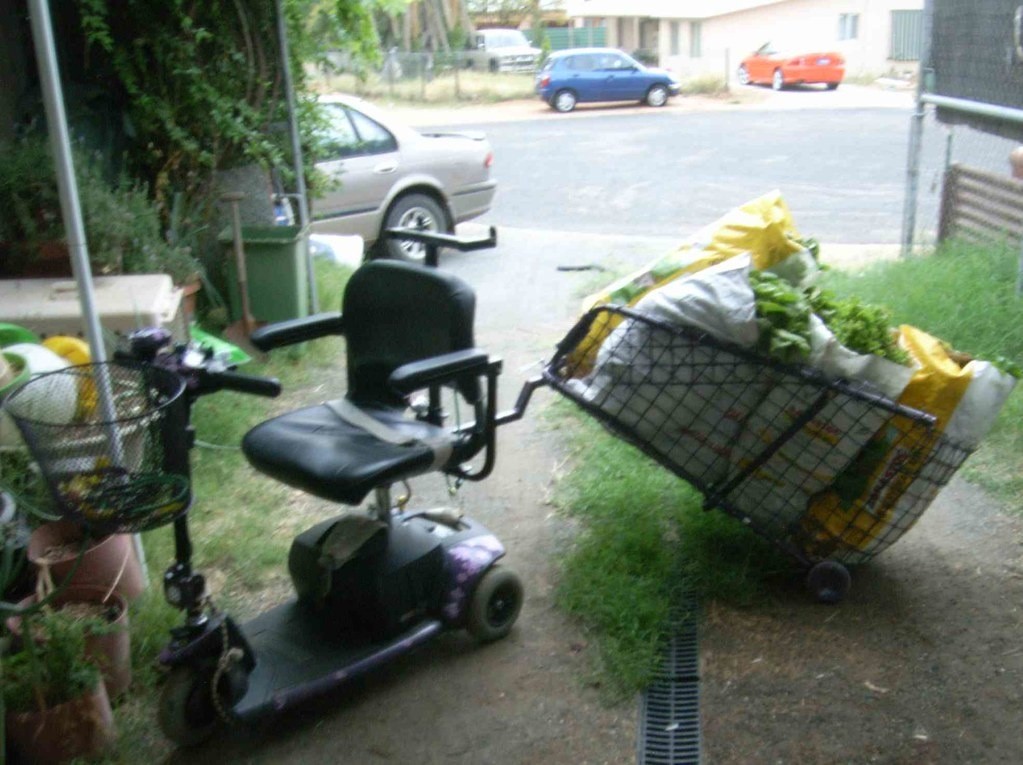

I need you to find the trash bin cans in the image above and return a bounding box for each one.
[217,221,310,363]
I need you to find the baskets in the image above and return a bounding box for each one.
[4,361,194,535]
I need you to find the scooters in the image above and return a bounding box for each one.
[1,223,553,756]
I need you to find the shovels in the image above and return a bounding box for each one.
[222,192,270,365]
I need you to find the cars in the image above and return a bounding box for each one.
[736,38,846,92]
[296,91,497,266]
[532,47,681,113]
[475,28,542,72]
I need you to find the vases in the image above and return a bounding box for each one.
[27,512,148,603]
[5,585,132,706]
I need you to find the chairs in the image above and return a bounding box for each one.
[241,255,505,529]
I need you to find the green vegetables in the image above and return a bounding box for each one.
[750,269,908,366]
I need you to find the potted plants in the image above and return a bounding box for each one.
[0,604,127,765]
